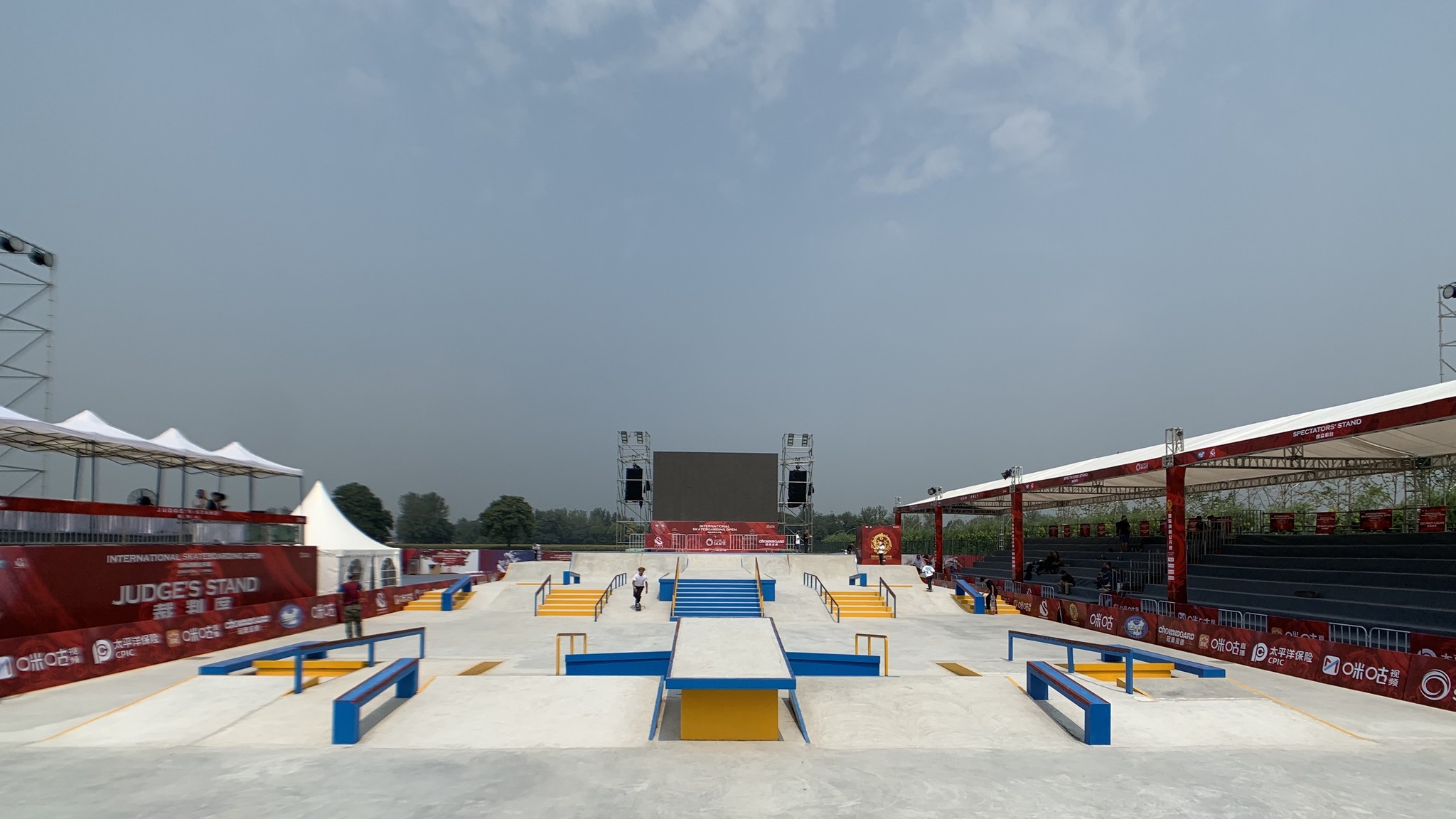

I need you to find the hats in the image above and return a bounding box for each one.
[1060,570,1066,574]
[1208,515,1215,520]
[1196,516,1202,520]
[1123,517,1127,520]
[879,542,882,545]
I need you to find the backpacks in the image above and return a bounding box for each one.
[1026,563,1034,572]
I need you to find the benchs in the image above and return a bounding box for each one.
[949,529,1456,655]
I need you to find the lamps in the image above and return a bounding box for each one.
[1000,467,1014,481]
[927,487,937,495]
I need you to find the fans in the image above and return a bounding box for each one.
[127,488,158,506]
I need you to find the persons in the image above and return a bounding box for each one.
[795,530,802,553]
[191,489,228,545]
[877,541,885,565]
[632,567,648,610]
[1057,570,1076,596]
[949,556,963,573]
[1335,516,1361,533]
[1391,510,1419,533]
[1237,520,1265,534]
[1093,561,1115,591]
[1192,514,1221,545]
[913,555,935,592]
[1118,515,1132,553]
[846,541,855,554]
[336,573,364,639]
[980,576,998,614]
[531,542,543,560]
[1031,550,1062,576]
[803,530,812,553]
[1447,509,1456,532]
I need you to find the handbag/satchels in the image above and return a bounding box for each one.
[1024,572,1032,581]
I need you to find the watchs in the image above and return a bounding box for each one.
[633,587,636,589]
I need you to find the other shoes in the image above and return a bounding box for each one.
[1031,568,1038,574]
[1104,584,1110,587]
[1063,593,1069,595]
[1056,592,1063,595]
[1099,588,1104,590]
[1093,581,1099,584]
[1105,588,1111,591]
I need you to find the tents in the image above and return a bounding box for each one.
[284,481,403,597]
[0,403,305,547]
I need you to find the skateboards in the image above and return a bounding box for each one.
[534,559,538,561]
[882,560,886,565]
[635,603,641,611]
[990,594,996,615]
[926,589,934,592]
